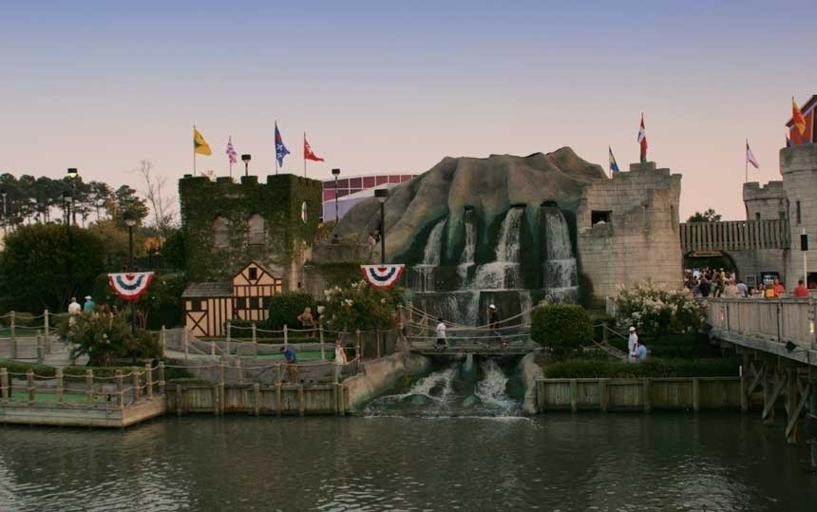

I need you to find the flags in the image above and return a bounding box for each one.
[225,141,236,163]
[785,136,791,152]
[608,148,618,172]
[746,142,759,170]
[274,126,290,168]
[636,117,647,160]
[791,97,806,136]
[304,138,324,162]
[193,129,211,155]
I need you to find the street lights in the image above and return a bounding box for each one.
[374,187,389,354]
[70,164,79,228]
[59,189,72,280]
[332,167,341,225]
[240,154,252,176]
[120,211,142,338]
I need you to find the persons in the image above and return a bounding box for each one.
[67,297,80,327]
[279,346,299,384]
[626,326,638,362]
[84,295,95,313]
[631,340,646,361]
[487,304,508,347]
[333,339,346,383]
[296,306,316,338]
[433,317,447,352]
[684,267,808,300]
